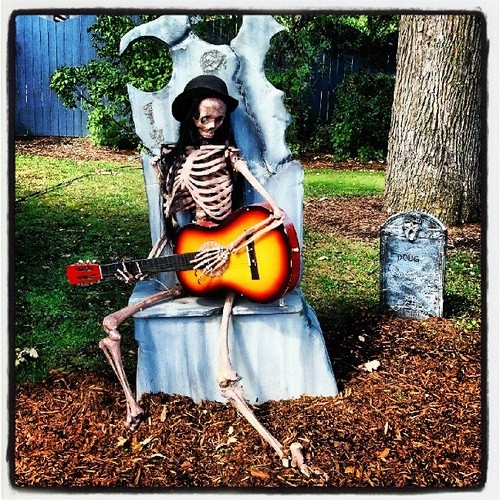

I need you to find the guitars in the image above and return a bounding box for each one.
[67,202,301,304]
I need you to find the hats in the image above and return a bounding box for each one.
[169,75,240,120]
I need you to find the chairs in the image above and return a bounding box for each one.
[121,12,340,408]
